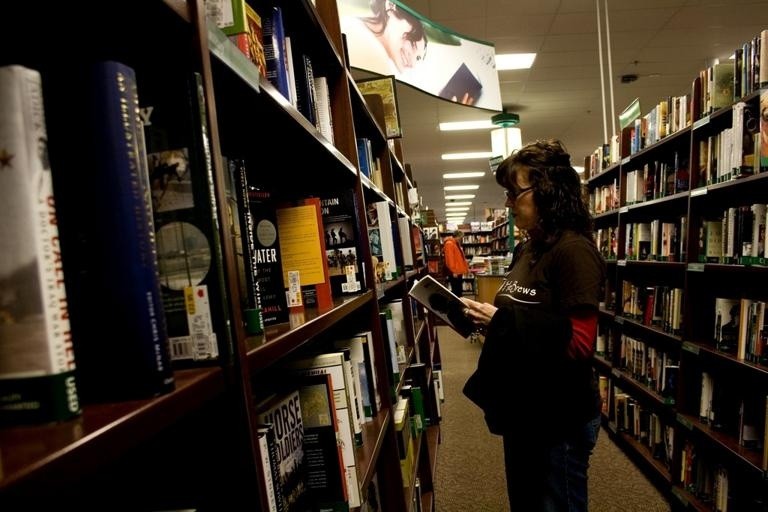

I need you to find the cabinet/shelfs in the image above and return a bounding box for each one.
[582,78,767,511]
[2,0,445,512]
[460,220,534,298]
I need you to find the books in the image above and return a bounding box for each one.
[0,0,768,511]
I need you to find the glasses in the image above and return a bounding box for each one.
[503,186,535,199]
